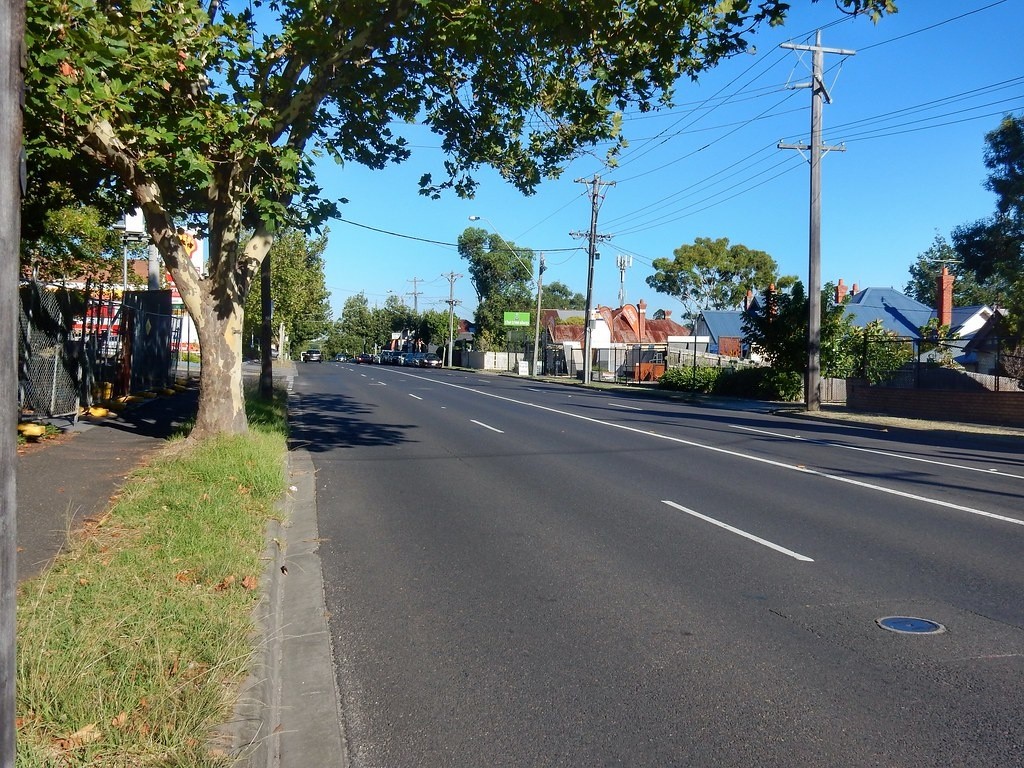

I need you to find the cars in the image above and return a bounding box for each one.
[334,350,442,370]
[303,349,324,364]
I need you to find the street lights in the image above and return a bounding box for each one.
[468,215,544,378]
